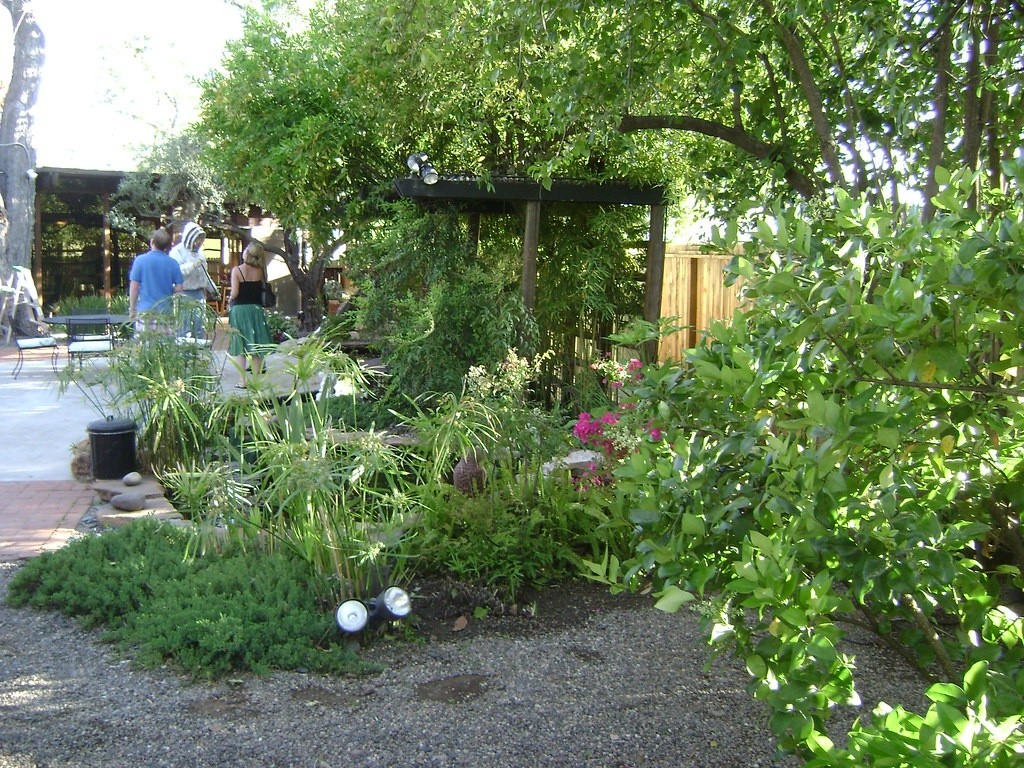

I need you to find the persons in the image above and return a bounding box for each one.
[128,222,222,398]
[228,242,273,389]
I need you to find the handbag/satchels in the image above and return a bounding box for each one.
[261,269,276,307]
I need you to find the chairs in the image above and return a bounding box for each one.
[6,313,60,380]
[65,317,114,365]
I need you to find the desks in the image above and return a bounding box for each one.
[41,313,133,371]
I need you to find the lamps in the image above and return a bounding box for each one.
[407,152,441,184]
[331,583,413,644]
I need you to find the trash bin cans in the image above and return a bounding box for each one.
[85,415,138,481]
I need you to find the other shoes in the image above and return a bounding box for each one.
[235,383,247,389]
